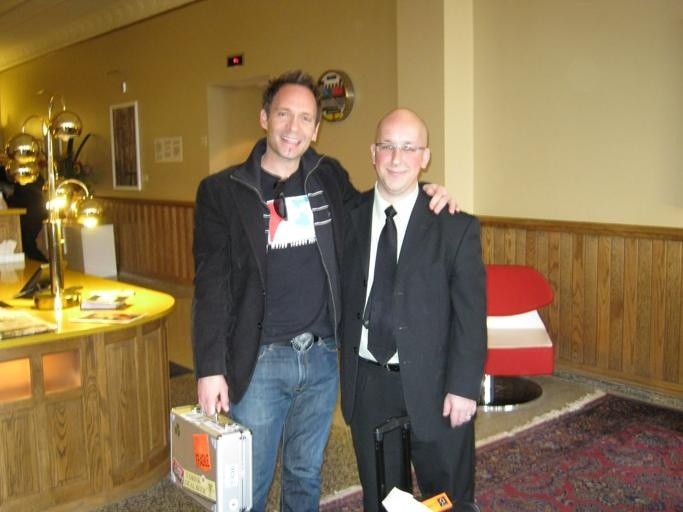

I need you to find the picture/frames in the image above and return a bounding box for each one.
[108,99,141,192]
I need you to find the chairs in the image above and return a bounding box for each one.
[476,262,556,412]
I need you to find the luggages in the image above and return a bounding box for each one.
[375,416,480,511]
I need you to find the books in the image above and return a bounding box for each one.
[0,312,50,339]
[81,296,129,310]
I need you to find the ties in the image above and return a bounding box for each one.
[363,206,398,365]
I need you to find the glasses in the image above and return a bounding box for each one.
[375,142,424,152]
[273,178,288,221]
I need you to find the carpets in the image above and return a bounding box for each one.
[317,387,683,512]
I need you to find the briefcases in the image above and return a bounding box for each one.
[170,405,253,512]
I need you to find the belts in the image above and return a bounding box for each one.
[373,363,399,372]
[274,333,318,352]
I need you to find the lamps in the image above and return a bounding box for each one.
[3,93,105,312]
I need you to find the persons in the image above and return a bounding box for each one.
[193,72,460,512]
[340,109,487,512]
[0,154,48,263]
